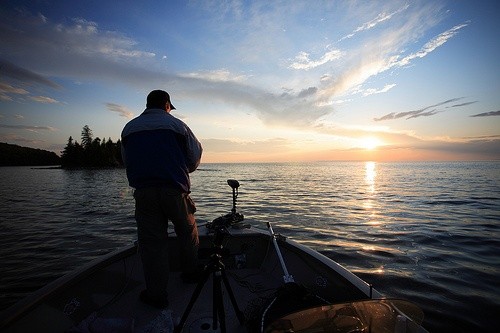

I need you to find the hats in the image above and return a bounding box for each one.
[146,90,177,110]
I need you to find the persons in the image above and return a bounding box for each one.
[120,89,204,275]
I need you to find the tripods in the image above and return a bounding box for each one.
[173,231,245,333]
